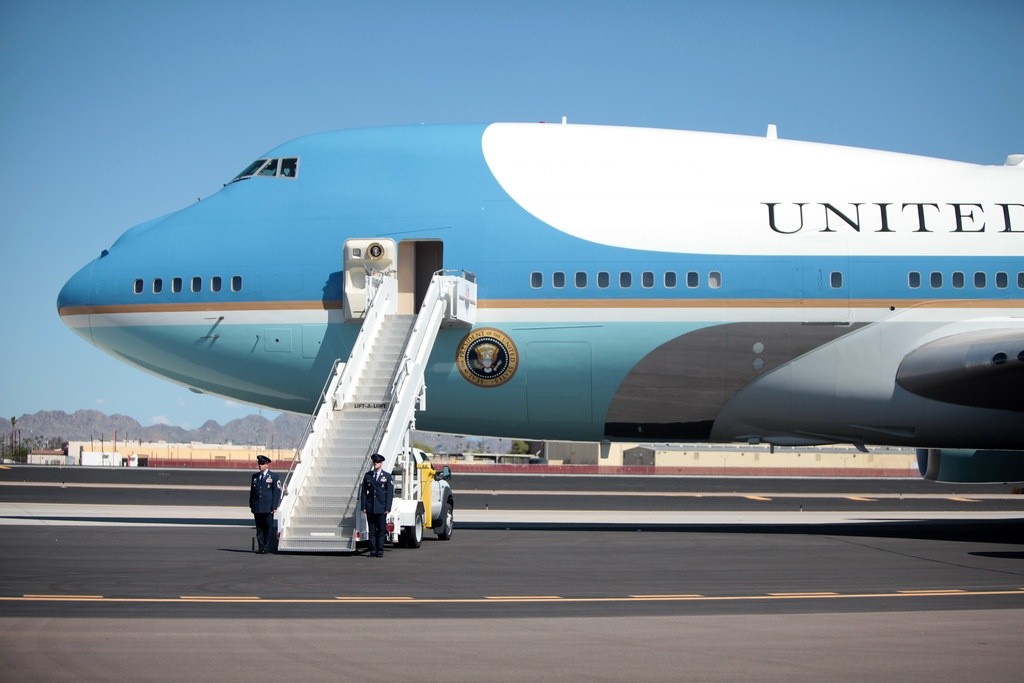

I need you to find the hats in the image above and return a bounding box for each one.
[257,455,271,464]
[371,454,385,462]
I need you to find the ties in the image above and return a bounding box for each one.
[260,472,264,484]
[373,473,378,482]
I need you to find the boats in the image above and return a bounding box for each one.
[51,119,1024,482]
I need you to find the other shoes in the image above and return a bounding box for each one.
[376,553,383,557]
[367,553,374,558]
[262,549,270,554]
[255,549,262,554]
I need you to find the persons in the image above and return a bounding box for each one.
[249,455,281,554]
[360,454,393,558]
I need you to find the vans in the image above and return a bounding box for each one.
[400,447,454,548]
